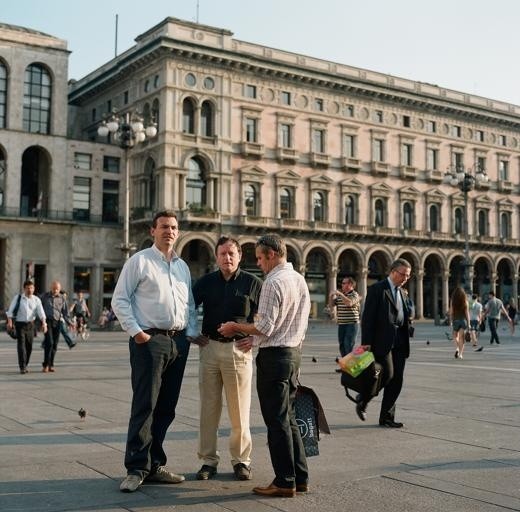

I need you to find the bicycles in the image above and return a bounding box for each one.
[69,313,91,342]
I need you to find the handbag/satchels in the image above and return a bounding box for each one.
[6,319,19,339]
[341,362,382,398]
[292,383,330,457]
[338,346,376,378]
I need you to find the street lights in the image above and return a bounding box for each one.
[95,104,163,263]
[441,161,491,295]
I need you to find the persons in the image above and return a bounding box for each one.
[40,280,65,373]
[403,288,416,320]
[356,257,413,428]
[449,286,470,359]
[215,232,312,497]
[109,209,200,493]
[328,277,360,374]
[445,290,519,347]
[41,290,115,351]
[6,280,48,374]
[186,237,265,479]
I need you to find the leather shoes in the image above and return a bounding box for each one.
[252,479,297,498]
[379,418,403,429]
[296,481,309,492]
[356,393,368,421]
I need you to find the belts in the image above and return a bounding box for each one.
[202,332,249,344]
[143,328,186,337]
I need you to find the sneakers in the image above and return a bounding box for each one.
[233,463,251,481]
[454,340,502,360]
[119,474,146,492]
[19,365,56,374]
[196,464,217,480]
[146,465,186,484]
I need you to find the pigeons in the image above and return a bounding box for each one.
[78,407,87,421]
